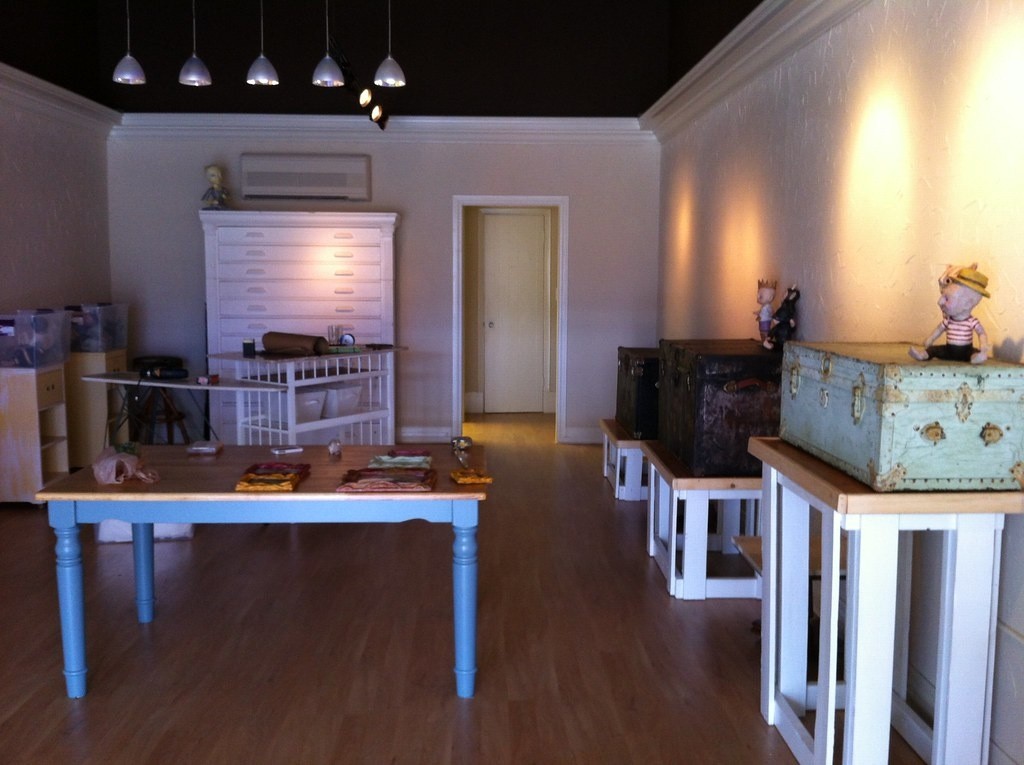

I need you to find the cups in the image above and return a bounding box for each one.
[243,341,255,358]
[328,325,341,346]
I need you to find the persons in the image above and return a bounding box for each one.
[762,286,800,351]
[200,165,231,211]
[907,260,990,364]
[751,279,776,343]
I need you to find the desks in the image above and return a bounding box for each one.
[747,433,1024,765]
[599,418,650,501]
[639,442,763,601]
[34,442,489,700]
[81,369,287,444]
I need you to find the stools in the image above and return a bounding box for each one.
[730,533,848,679]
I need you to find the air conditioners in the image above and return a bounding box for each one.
[240,154,372,201]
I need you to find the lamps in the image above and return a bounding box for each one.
[374,0,406,88]
[312,0,345,86]
[179,0,212,86]
[246,0,280,85]
[113,0,146,85]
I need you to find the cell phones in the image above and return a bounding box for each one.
[271,446,303,455]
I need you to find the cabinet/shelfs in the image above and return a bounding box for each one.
[206,343,409,447]
[65,346,129,468]
[0,361,69,509]
[199,211,399,444]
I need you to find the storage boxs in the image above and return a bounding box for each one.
[657,337,782,477]
[775,341,1024,490]
[0,303,129,369]
[615,346,657,439]
[265,381,362,424]
